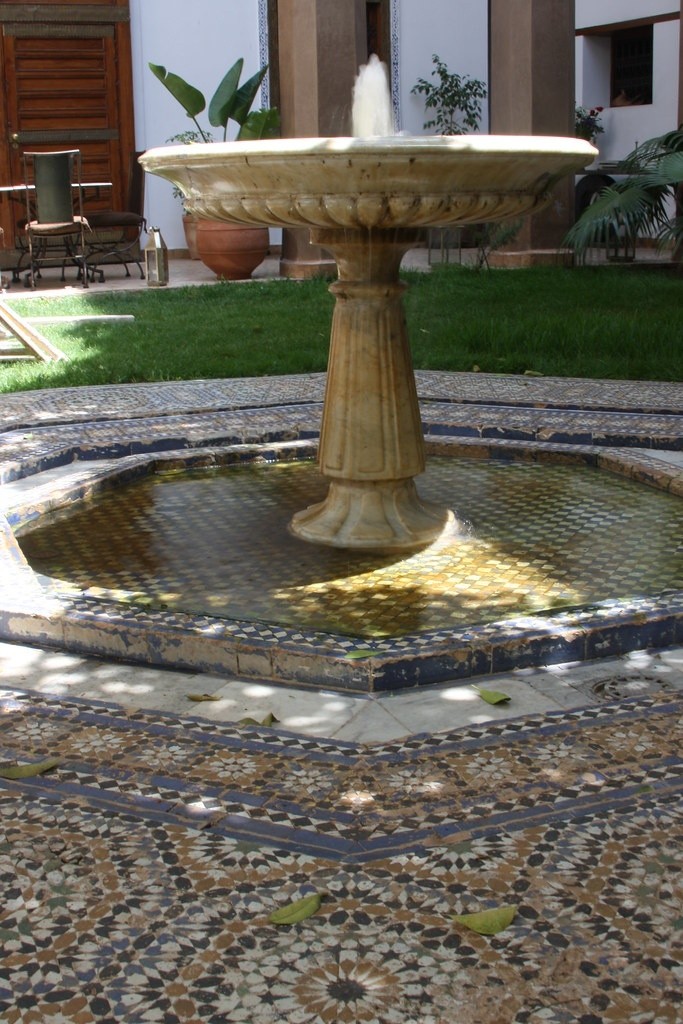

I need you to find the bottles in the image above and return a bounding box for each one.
[144,226,169,287]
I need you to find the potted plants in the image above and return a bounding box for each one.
[147,57,280,279]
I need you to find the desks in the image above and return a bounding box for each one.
[0,182,112,287]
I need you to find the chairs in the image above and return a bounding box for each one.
[23,149,90,289]
[84,150,147,282]
